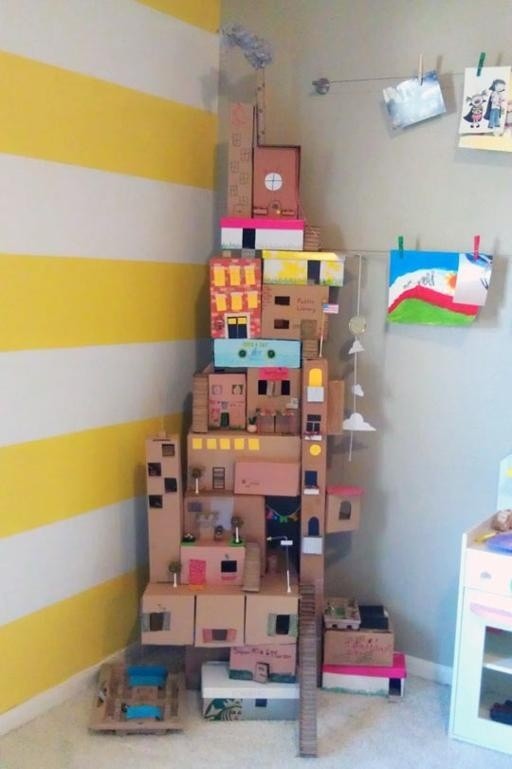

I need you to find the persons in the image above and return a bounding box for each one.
[463,88,485,129]
[486,79,505,128]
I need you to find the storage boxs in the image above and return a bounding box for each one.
[325,485,365,534]
[191,249,345,435]
[303,225,321,251]
[298,582,319,756]
[87,663,189,737]
[322,593,407,696]
[143,425,327,725]
[217,101,305,251]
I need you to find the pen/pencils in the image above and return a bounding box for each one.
[475,531,498,542]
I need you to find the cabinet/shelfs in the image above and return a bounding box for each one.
[449,507,512,756]
